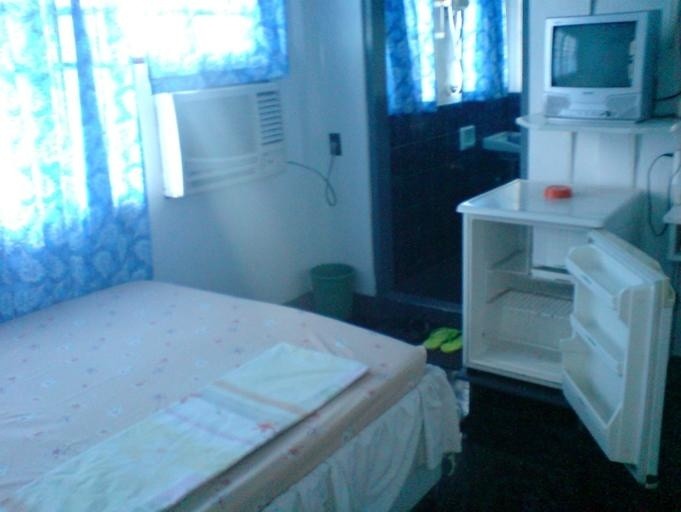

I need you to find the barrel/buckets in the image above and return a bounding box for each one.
[309,261,359,320]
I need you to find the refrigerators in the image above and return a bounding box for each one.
[456,179,677,491]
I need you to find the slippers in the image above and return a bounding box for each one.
[422,325,458,354]
[440,331,462,354]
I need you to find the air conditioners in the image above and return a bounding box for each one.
[154,82,286,198]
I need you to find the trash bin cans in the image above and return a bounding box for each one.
[311,263,354,320]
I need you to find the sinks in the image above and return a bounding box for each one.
[481,129,523,153]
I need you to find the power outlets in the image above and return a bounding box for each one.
[329,133,341,156]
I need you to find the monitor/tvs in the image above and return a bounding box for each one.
[543,10,658,121]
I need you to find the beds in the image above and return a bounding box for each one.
[0,280,447,512]
